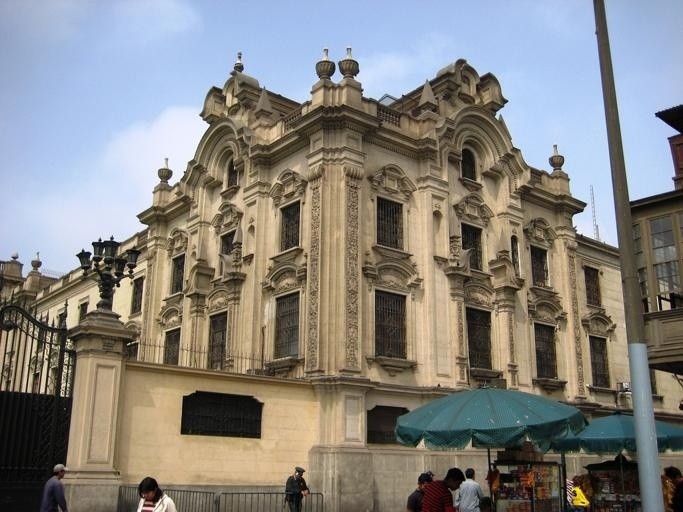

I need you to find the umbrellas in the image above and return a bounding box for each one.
[550,413,683,512]
[394,383,591,512]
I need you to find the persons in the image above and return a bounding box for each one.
[136,477,177,512]
[453,468,484,512]
[479,496,494,512]
[664,466,683,511]
[418,468,465,512]
[40,464,68,512]
[407,472,433,512]
[571,475,593,511]
[285,467,310,512]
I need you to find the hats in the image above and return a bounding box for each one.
[54,464,68,473]
[297,467,305,475]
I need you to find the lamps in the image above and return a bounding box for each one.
[75,235,142,309]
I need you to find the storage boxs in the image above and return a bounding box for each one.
[497,441,543,462]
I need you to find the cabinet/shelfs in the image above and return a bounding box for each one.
[489,462,563,511]
